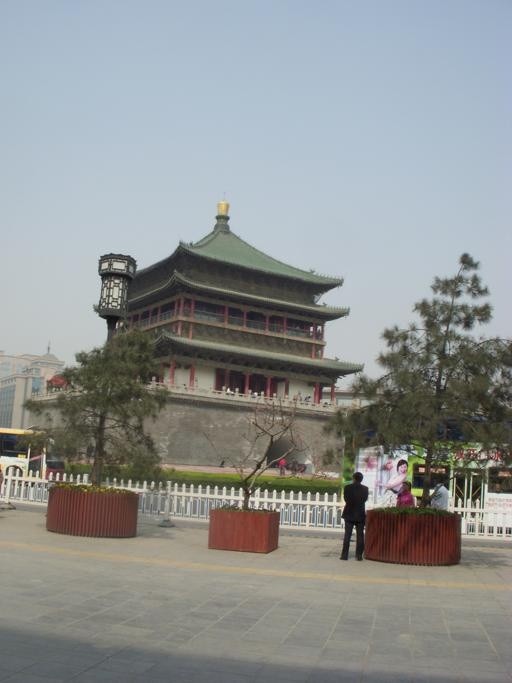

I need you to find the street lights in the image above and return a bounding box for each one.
[90,252,136,487]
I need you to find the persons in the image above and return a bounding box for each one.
[278,457,286,474]
[289,456,298,475]
[429,471,449,511]
[375,456,408,508]
[396,480,416,509]
[340,471,368,561]
[290,390,331,410]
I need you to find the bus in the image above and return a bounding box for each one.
[0,428,47,484]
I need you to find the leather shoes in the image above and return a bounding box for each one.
[357,556,363,561]
[339,557,348,560]
